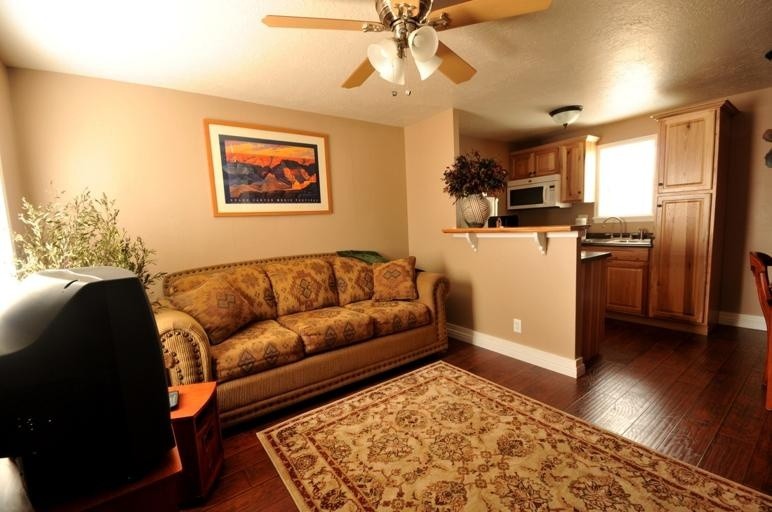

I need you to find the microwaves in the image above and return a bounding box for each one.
[506,173,573,209]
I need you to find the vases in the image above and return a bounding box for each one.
[461,192,491,229]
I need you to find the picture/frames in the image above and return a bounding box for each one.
[203,117,336,219]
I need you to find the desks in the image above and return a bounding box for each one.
[73,422,184,512]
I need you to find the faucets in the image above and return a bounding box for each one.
[601,216,623,239]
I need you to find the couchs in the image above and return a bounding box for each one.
[149,250,453,431]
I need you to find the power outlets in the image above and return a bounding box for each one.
[513,319,522,333]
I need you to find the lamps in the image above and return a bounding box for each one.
[365,21,444,88]
[548,104,587,127]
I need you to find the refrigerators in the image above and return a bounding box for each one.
[462,196,499,227]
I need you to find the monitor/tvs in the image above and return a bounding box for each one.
[0,266,176,512]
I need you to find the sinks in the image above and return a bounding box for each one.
[590,238,610,244]
[620,238,640,243]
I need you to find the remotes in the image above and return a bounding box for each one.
[169,391,179,409]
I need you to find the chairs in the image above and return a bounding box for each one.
[750,252,772,412]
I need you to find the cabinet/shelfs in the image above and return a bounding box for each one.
[605,259,649,318]
[559,142,585,203]
[167,381,225,507]
[509,148,558,181]
[649,193,711,325]
[658,109,716,193]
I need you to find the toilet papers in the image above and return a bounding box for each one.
[574,218,586,226]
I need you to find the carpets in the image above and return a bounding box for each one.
[251,357,772,512]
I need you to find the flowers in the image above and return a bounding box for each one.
[439,148,508,205]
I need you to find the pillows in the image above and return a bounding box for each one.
[196,266,277,321]
[264,258,340,317]
[326,254,374,307]
[164,274,255,345]
[371,255,419,303]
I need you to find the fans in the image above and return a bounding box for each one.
[259,0,551,90]
